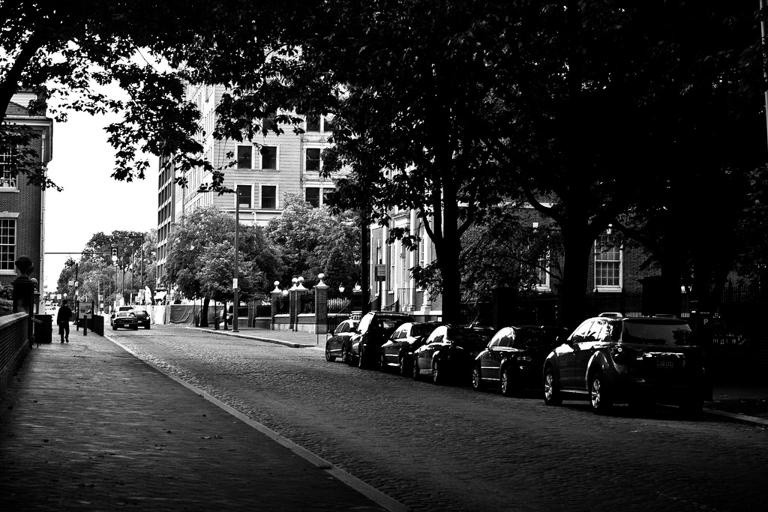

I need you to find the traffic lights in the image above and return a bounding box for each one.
[110,243,119,263]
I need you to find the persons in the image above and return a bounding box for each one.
[57,299,72,344]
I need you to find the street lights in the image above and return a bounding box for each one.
[197,189,243,332]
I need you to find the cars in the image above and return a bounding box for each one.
[111,306,150,330]
